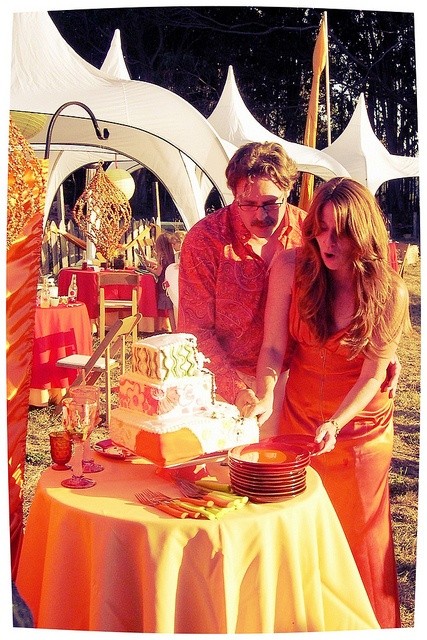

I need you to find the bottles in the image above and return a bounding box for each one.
[41,275,50,308]
[68,274,78,305]
[82,250,88,270]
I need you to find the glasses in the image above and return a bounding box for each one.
[236,196,285,211]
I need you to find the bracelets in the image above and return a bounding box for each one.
[326,418,341,437]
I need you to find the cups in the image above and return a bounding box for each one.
[51,297,59,307]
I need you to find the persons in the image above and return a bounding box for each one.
[179,142,403,444]
[170,231,185,253]
[240,177,403,630]
[144,233,177,334]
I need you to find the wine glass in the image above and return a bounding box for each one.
[70,386,104,473]
[61,397,98,489]
[49,431,74,471]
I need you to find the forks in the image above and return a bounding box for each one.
[134,472,249,521]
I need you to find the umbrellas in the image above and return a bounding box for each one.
[32,28,240,235]
[321,92,419,195]
[13,10,237,233]
[203,61,352,182]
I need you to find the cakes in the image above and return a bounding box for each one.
[110,333,261,469]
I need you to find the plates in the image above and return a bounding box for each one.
[227,433,326,502]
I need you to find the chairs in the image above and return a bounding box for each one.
[48,313,142,427]
[98,271,141,308]
[99,288,137,372]
